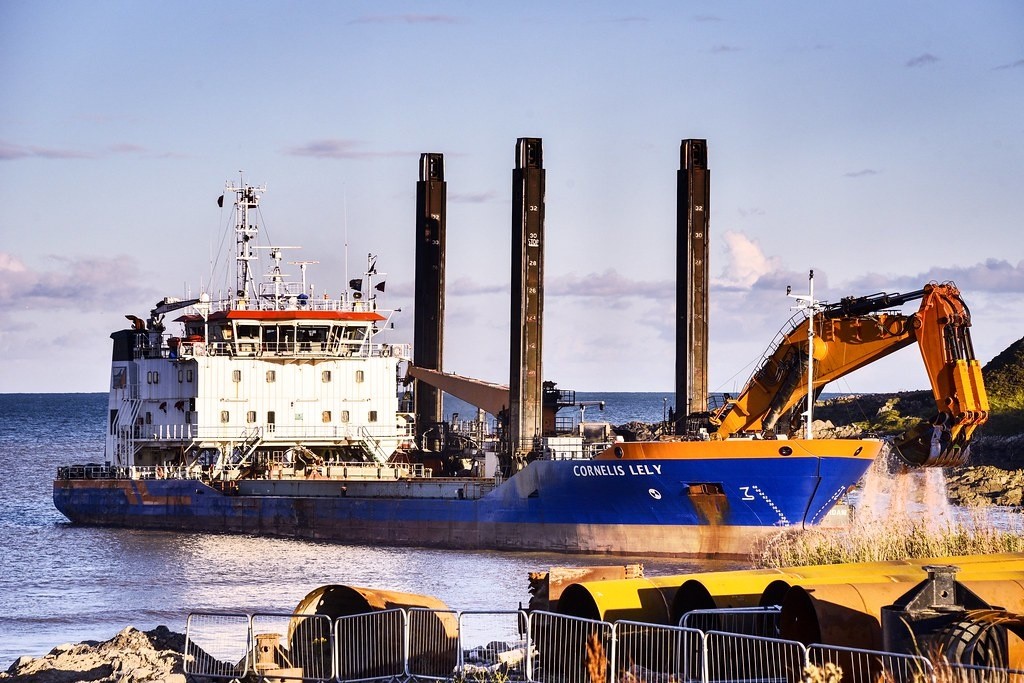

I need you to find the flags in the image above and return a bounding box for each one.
[375,281,385,292]
[349,279,362,291]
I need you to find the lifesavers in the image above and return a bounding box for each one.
[346,351,352,357]
[256,351,263,357]
[210,348,217,356]
[383,350,389,357]
[185,349,192,355]
[158,468,163,477]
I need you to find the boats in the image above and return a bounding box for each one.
[52,139,991,556]
